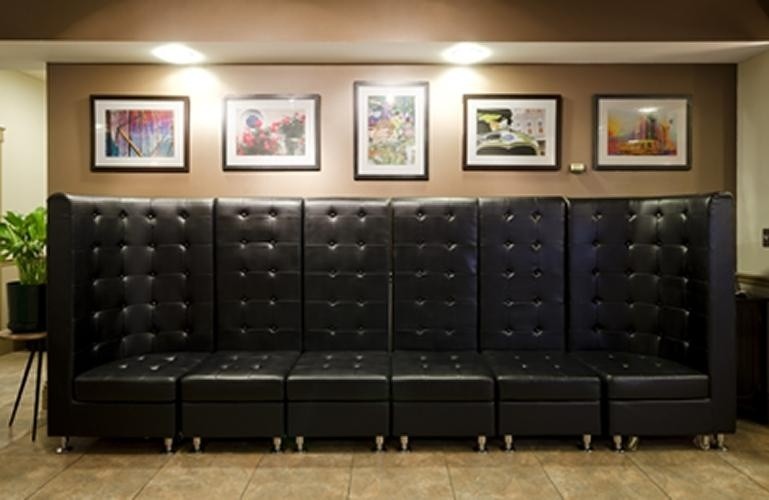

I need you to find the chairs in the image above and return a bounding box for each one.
[47,191,738,454]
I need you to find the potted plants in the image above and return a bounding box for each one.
[0,206,49,334]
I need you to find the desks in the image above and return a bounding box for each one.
[8,338,49,442]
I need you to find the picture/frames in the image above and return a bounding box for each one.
[462,93,563,172]
[591,91,693,172]
[219,94,321,173]
[353,80,430,183]
[88,92,191,174]
